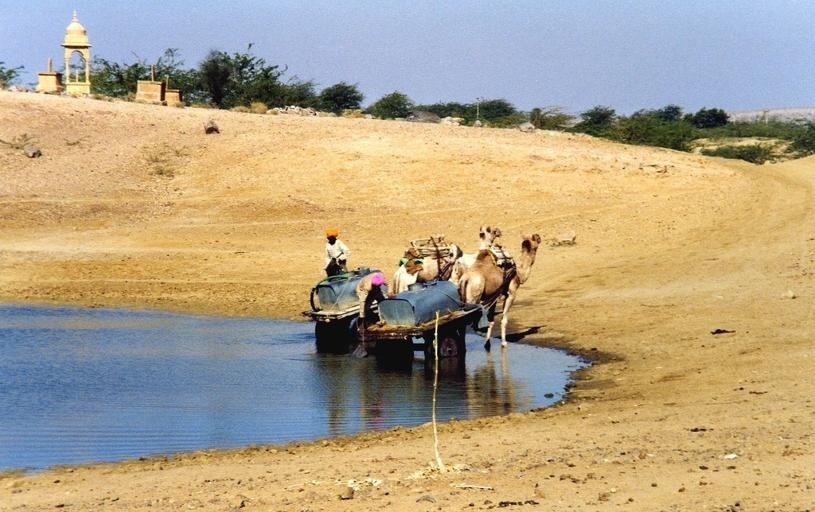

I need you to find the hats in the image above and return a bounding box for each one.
[372,272,384,286]
[327,230,338,237]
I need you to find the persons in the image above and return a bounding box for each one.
[324,227,352,273]
[351,271,390,358]
